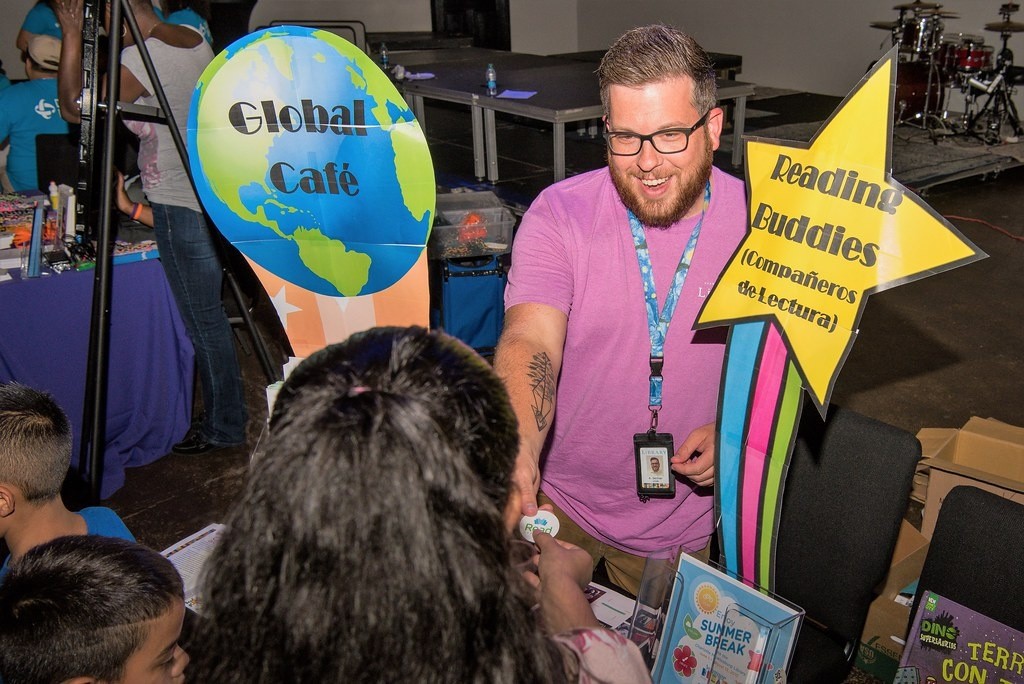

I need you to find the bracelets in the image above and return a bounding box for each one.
[129,202,143,220]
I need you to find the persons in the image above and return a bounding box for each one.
[649,458,663,476]
[0,534,190,684]
[0,381,137,582]
[17,0,62,53]
[493,24,747,597]
[186,325,653,684]
[167,0,211,45]
[0,34,69,193]
[53,0,249,454]
[115,170,154,228]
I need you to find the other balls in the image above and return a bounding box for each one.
[519,510,561,543]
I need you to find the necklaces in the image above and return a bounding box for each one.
[147,23,157,35]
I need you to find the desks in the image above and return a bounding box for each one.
[382,49,754,191]
[0,189,196,500]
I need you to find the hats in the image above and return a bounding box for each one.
[28,35,63,70]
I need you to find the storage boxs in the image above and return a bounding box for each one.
[851,415,1024,683]
[427,190,517,261]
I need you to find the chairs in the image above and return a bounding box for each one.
[35,134,83,195]
[906,484,1024,646]
[707,391,923,684]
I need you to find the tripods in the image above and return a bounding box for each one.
[896,9,1024,146]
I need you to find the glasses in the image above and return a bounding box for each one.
[602,110,711,156]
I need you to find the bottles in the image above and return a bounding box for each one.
[48,182,60,209]
[486,63,497,95]
[380,42,389,70]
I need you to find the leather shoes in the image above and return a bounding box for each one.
[171,432,225,456]
[189,413,205,431]
